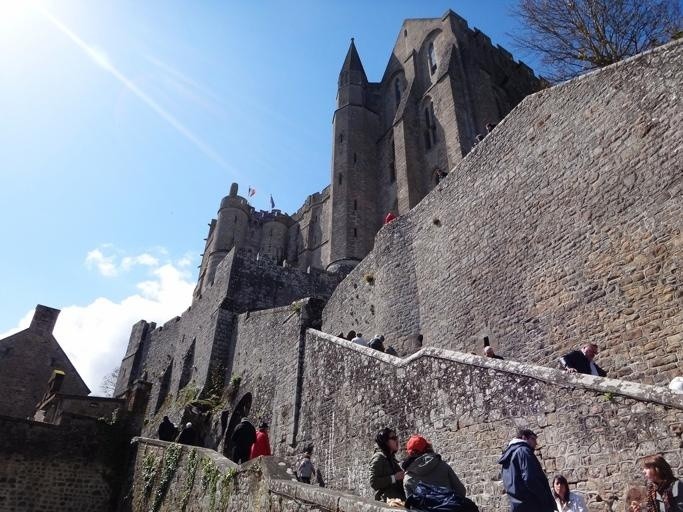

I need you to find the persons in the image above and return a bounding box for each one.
[496,428,558,511]
[470,123,494,150]
[274,255,292,268]
[230,417,256,465]
[385,209,396,224]
[337,330,398,357]
[632,455,683,512]
[559,344,605,378]
[400,436,479,511]
[436,169,447,185]
[483,346,503,361]
[298,454,315,484]
[248,421,271,462]
[367,428,406,503]
[551,475,589,512]
[159,415,199,447]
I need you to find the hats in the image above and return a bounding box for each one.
[242,417,249,422]
[406,439,432,455]
[259,423,267,427]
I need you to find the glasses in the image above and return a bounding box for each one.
[389,434,398,440]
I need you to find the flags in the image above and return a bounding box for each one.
[270,197,275,209]
[249,186,255,197]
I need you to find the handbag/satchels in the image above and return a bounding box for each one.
[408,482,464,511]
